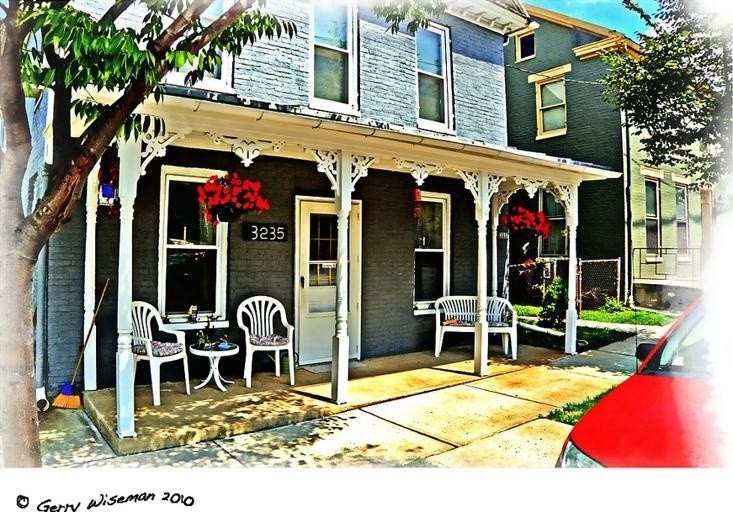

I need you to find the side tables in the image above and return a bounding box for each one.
[189,340,240,392]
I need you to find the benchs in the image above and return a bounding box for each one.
[432,295,518,362]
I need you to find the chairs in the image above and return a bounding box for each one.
[128,300,191,407]
[236,294,297,389]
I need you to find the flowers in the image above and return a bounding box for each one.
[500,204,552,244]
[197,169,272,227]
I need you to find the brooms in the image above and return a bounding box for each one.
[51,277,112,409]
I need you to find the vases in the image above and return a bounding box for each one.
[217,203,245,225]
[516,227,536,244]
